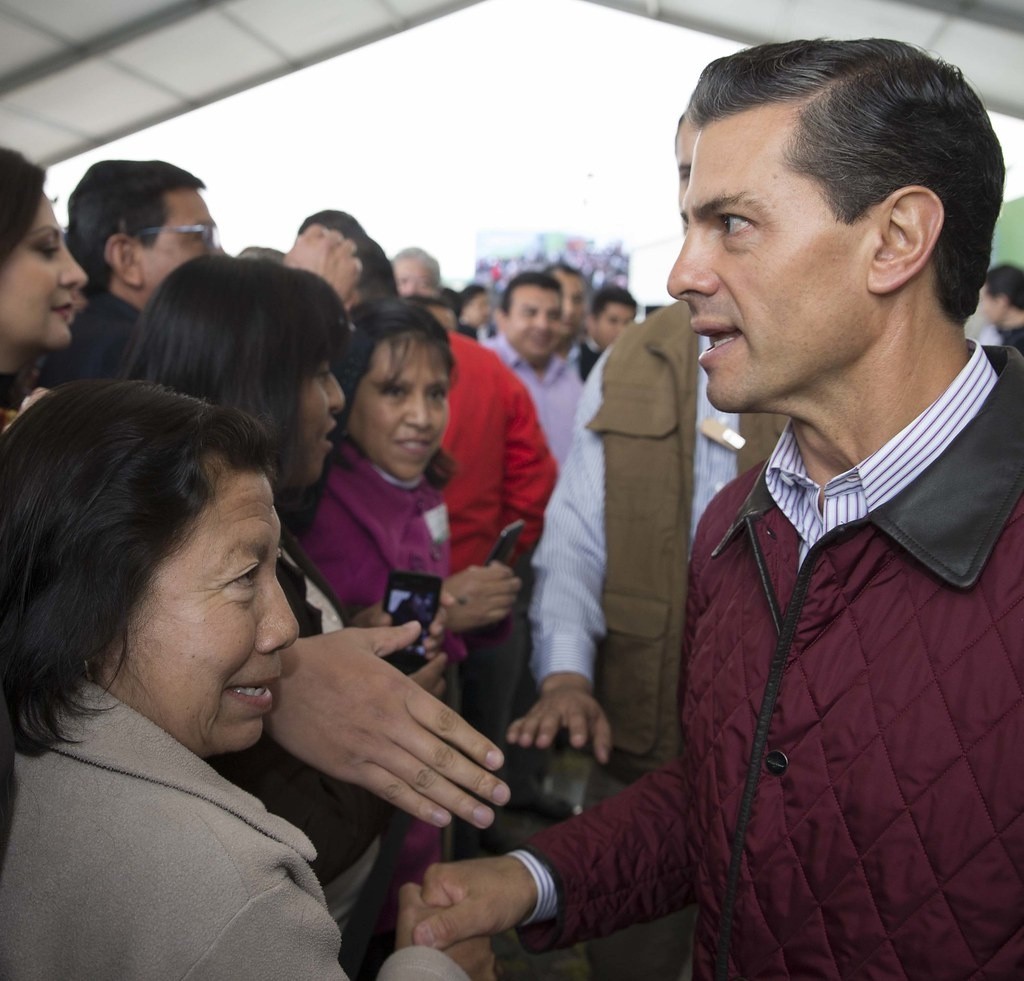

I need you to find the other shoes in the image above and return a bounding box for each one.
[504,790,580,827]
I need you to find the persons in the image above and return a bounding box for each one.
[0,148,509,981]
[418,38,1024,981]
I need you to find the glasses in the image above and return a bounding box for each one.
[126,224,214,250]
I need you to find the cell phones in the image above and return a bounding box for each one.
[483,519,525,566]
[380,570,443,675]
